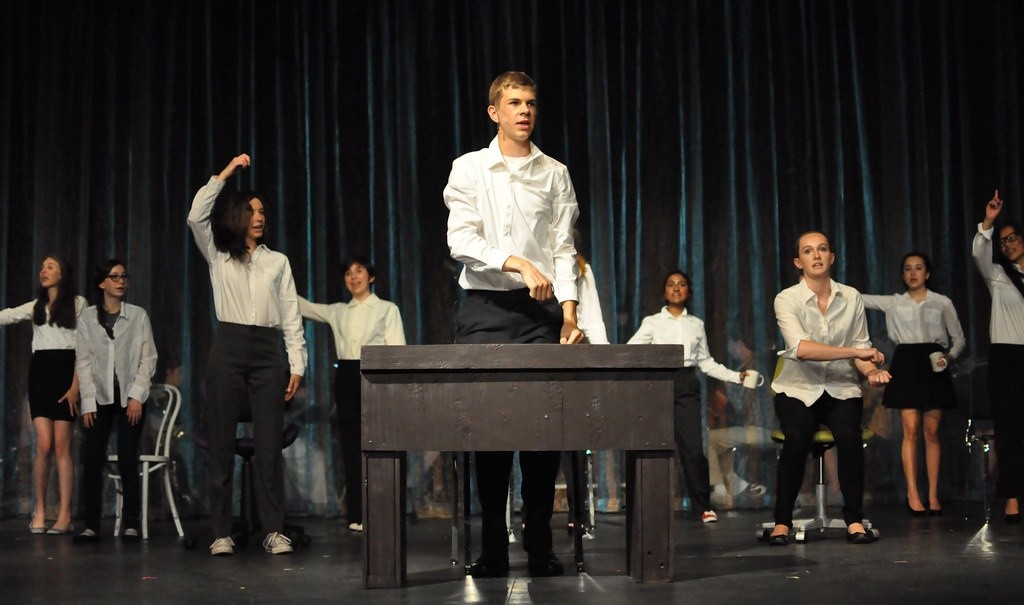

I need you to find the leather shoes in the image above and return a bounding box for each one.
[463,562,509,578]
[529,556,563,575]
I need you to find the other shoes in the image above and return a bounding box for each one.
[847,527,871,543]
[72,534,99,543]
[929,504,943,515]
[123,534,140,542]
[769,528,790,545]
[1003,503,1021,522]
[29,521,45,534]
[349,523,363,531]
[46,521,74,535]
[904,497,927,518]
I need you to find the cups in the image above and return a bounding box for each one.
[743,369,764,389]
[929,352,947,372]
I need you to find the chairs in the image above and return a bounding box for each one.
[183,381,311,551]
[105,383,185,541]
[962,365,999,526]
[756,353,881,543]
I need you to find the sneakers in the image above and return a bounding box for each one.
[262,531,293,554]
[701,510,717,523]
[209,536,234,556]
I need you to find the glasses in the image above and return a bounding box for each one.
[104,275,128,282]
[999,232,1017,247]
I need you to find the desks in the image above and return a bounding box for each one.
[360,345,684,590]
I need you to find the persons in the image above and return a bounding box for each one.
[624,269,749,523]
[705,322,776,510]
[767,231,892,545]
[567,229,613,536]
[187,152,309,557]
[298,255,408,532]
[442,70,585,579]
[0,254,89,536]
[74,260,158,544]
[860,250,965,517]
[137,357,204,497]
[971,189,1024,524]
